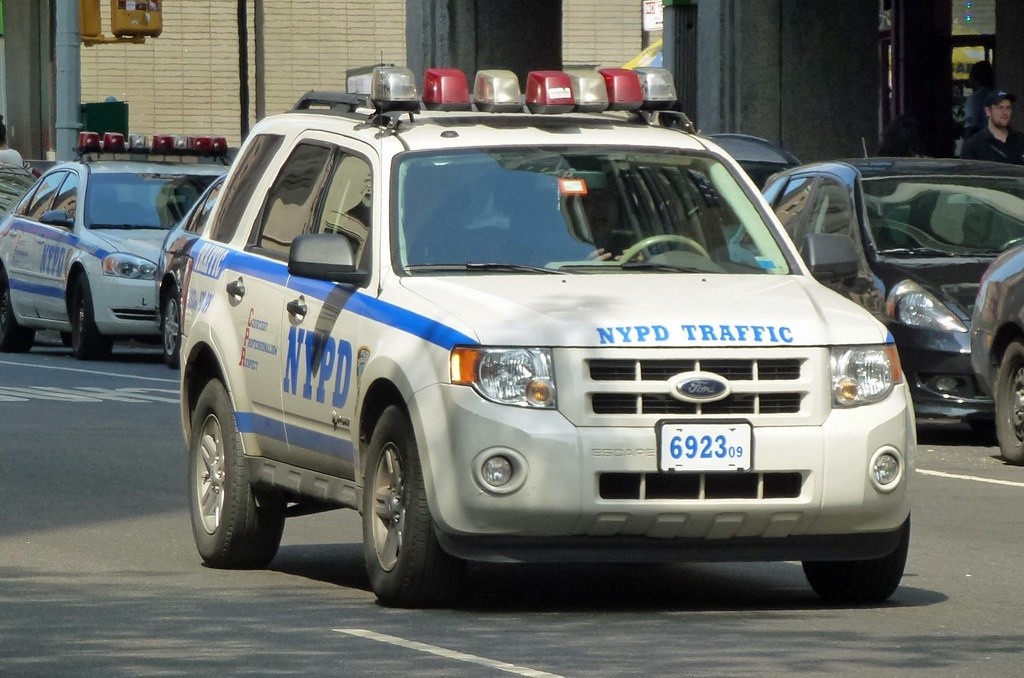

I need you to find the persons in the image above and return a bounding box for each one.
[951,61,993,157]
[872,117,948,246]
[962,90,1024,246]
[0,123,23,167]
[531,185,645,268]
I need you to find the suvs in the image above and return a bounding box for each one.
[178,66,916,608]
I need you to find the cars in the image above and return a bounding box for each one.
[0,131,235,361]
[970,245,1024,466]
[727,157,1024,431]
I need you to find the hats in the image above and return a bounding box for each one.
[985,90,1018,106]
